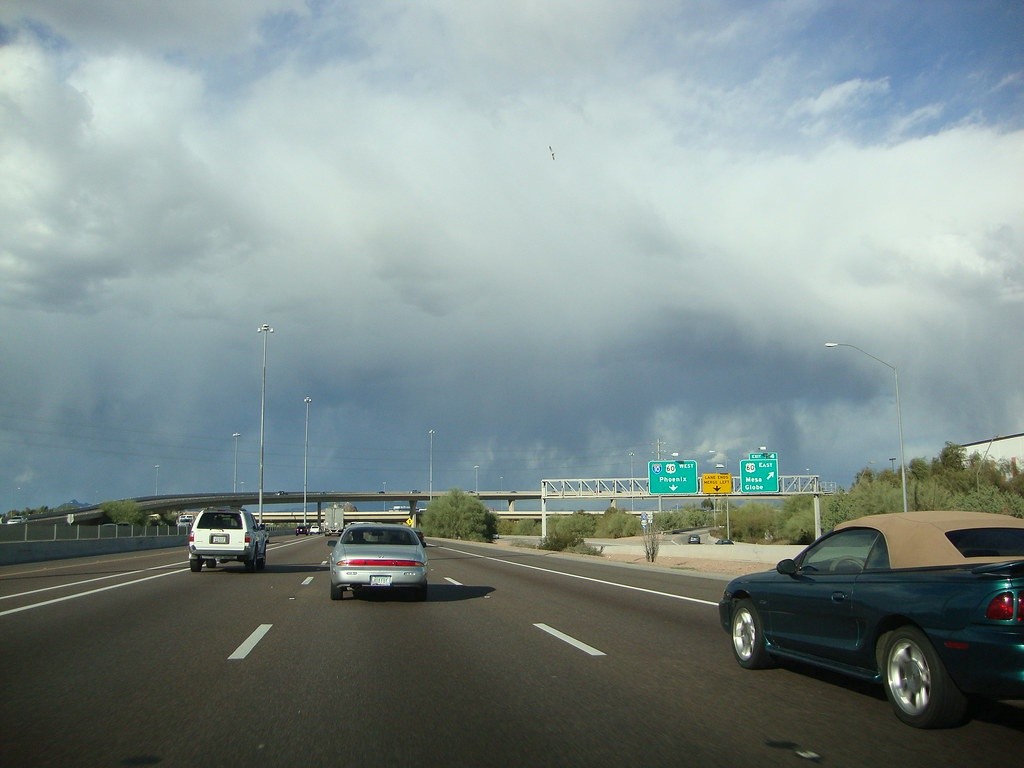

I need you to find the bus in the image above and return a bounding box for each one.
[393,506,421,511]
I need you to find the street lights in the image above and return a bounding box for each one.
[888,458,896,469]
[629,451,635,478]
[11,487,21,519]
[429,430,435,502]
[154,464,160,495]
[708,449,730,539]
[498,476,504,511]
[257,323,274,529]
[381,481,387,512]
[303,394,309,525]
[473,464,480,491]
[822,340,908,513]
[232,432,242,493]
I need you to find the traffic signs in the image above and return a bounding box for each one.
[739,460,779,494]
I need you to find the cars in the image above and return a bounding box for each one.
[6,516,27,524]
[409,489,422,494]
[118,522,135,526]
[688,534,700,544]
[101,524,119,528]
[715,539,734,545]
[376,491,385,495]
[327,523,430,601]
[414,529,427,548]
[274,491,288,495]
[295,526,309,536]
[718,511,1024,731]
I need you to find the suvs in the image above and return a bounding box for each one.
[188,506,267,574]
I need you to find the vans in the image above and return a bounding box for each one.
[180,515,194,522]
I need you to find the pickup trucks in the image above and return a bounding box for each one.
[310,526,320,535]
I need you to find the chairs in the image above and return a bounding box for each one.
[350,533,367,543]
[388,534,404,544]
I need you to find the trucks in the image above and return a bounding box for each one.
[324,508,345,537]
